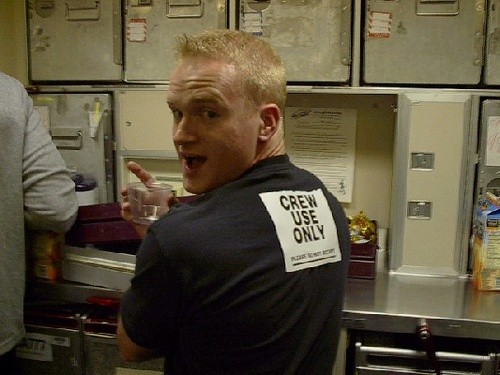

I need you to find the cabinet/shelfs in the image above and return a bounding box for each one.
[0,0,500,375]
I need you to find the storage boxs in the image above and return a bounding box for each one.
[63,246,136,290]
[66,196,196,244]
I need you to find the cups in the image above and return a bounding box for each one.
[126,182,173,226]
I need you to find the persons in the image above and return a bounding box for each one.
[0,72,81,375]
[116,28,350,375]
